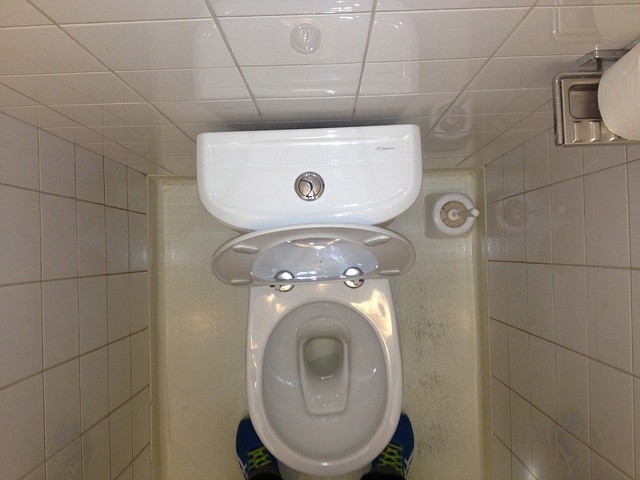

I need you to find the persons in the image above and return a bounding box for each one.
[235,412,414,480]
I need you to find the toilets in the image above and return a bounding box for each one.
[196,123,424,477]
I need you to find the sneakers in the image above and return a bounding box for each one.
[235,418,282,480]
[371,413,414,479]
